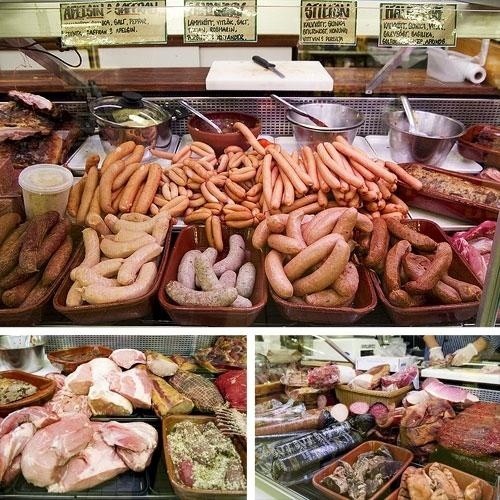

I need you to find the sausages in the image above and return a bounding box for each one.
[0,121,483,310]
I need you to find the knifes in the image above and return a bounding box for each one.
[269,92,330,129]
[251,53,286,80]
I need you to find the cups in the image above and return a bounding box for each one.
[18,163,74,220]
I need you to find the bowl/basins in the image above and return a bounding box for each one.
[185,111,262,156]
[283,103,366,158]
[455,124,498,168]
[380,110,467,170]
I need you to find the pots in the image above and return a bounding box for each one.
[86,89,188,166]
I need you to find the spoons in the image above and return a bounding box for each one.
[400,95,428,140]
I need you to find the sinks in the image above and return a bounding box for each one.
[369,44,428,67]
[297,45,369,63]
